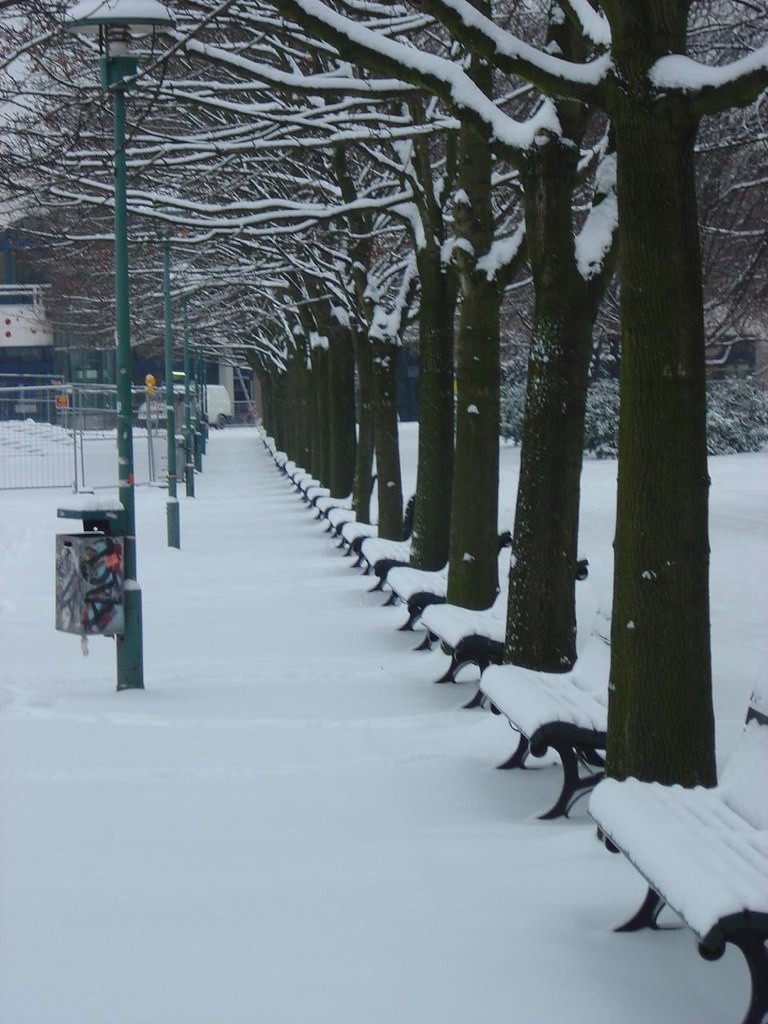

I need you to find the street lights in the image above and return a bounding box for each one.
[58,0,178,696]
[150,203,193,554]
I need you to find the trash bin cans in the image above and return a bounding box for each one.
[175,416,198,481]
[54,501,124,636]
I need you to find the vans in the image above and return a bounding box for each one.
[135,381,233,430]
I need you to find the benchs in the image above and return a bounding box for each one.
[255,423,767,1024]
